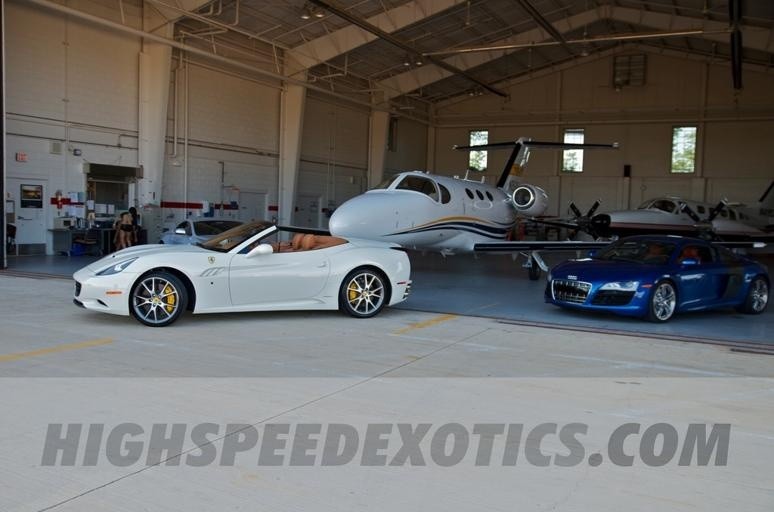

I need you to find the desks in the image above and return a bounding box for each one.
[47,225,148,257]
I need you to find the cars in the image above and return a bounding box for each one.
[155,217,254,254]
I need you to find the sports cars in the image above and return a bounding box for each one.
[543,233,771,323]
[72,218,413,328]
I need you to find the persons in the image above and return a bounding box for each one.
[119,206,138,249]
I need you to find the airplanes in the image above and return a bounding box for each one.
[269,137,621,280]
[524,177,774,259]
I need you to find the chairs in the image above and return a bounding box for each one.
[642,243,702,264]
[282,233,316,253]
